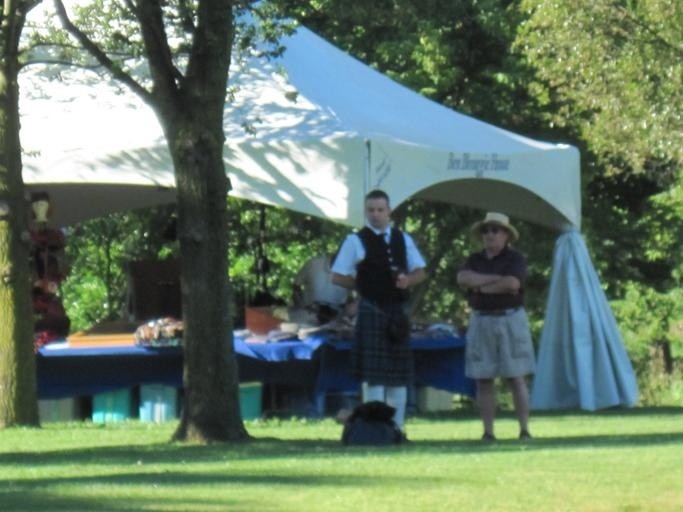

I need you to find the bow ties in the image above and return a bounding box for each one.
[376,232,388,244]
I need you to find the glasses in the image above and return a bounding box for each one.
[479,227,506,235]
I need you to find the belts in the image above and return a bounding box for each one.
[477,308,521,316]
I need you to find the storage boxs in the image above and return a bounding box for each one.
[236,379,265,422]
[34,394,83,429]
[416,383,455,416]
[137,381,180,425]
[90,386,134,427]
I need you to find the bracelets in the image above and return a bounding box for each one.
[476,286,481,295]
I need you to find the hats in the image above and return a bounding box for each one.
[472,212,519,243]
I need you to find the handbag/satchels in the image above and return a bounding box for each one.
[340,401,413,446]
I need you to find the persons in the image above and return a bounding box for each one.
[336,287,362,410]
[290,246,348,321]
[328,188,431,431]
[457,209,537,441]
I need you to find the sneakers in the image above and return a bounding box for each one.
[480,434,495,444]
[518,432,533,443]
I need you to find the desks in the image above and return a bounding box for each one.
[33,325,477,421]
